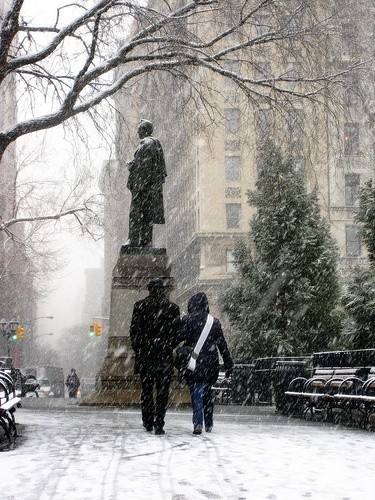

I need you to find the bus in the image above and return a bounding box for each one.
[310,348,374,389]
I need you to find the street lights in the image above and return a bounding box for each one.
[0,316,20,357]
[27,333,54,366]
[19,315,54,369]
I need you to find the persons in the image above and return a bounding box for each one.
[129,278,181,434]
[177,293,233,434]
[67,368,80,397]
[126,119,167,248]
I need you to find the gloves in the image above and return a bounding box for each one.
[220,358,234,378]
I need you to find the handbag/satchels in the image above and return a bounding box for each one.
[174,346,196,373]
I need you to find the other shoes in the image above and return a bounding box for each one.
[206,428,212,432]
[154,429,165,435]
[193,431,201,436]
[143,422,152,431]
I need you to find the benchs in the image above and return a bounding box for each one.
[281,366,375,433]
[0,371,23,443]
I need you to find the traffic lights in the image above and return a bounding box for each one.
[19,328,25,341]
[95,324,101,337]
[13,327,20,340]
[89,324,95,337]
